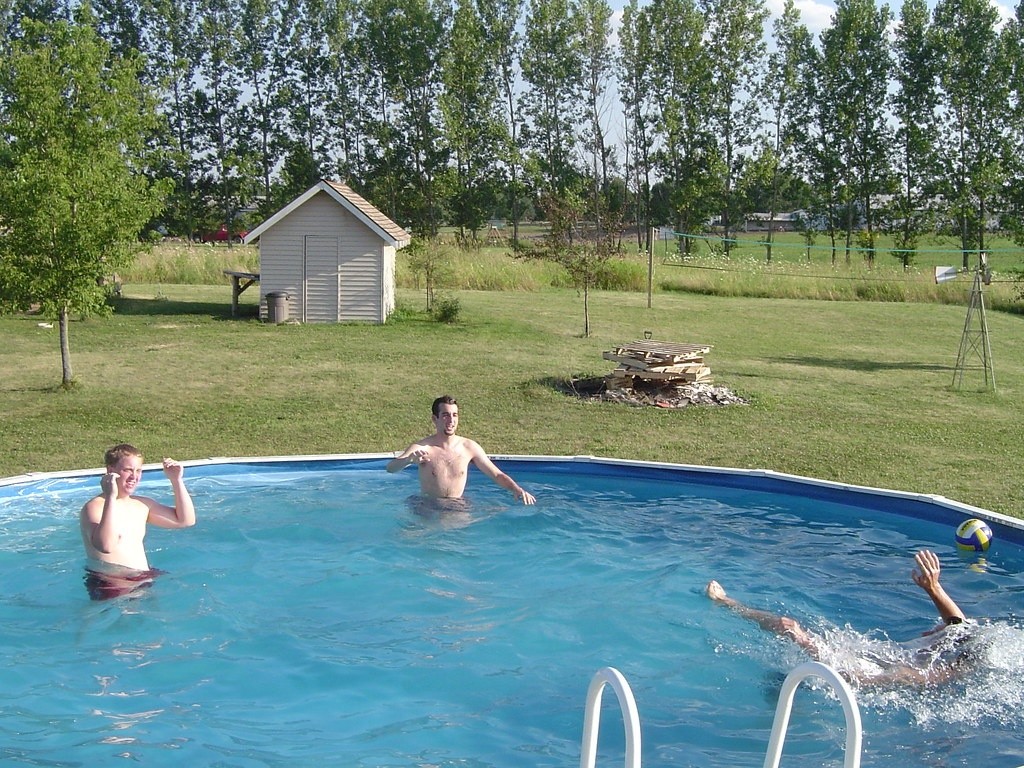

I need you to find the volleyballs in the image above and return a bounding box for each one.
[955,518,993,550]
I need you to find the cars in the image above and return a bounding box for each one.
[705,220,736,233]
[193,222,250,243]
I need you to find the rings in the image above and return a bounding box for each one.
[105,480,111,483]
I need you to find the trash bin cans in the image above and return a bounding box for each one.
[265,291,292,323]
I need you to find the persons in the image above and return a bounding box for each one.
[385,396,536,525]
[80,443,195,602]
[703,550,983,689]
